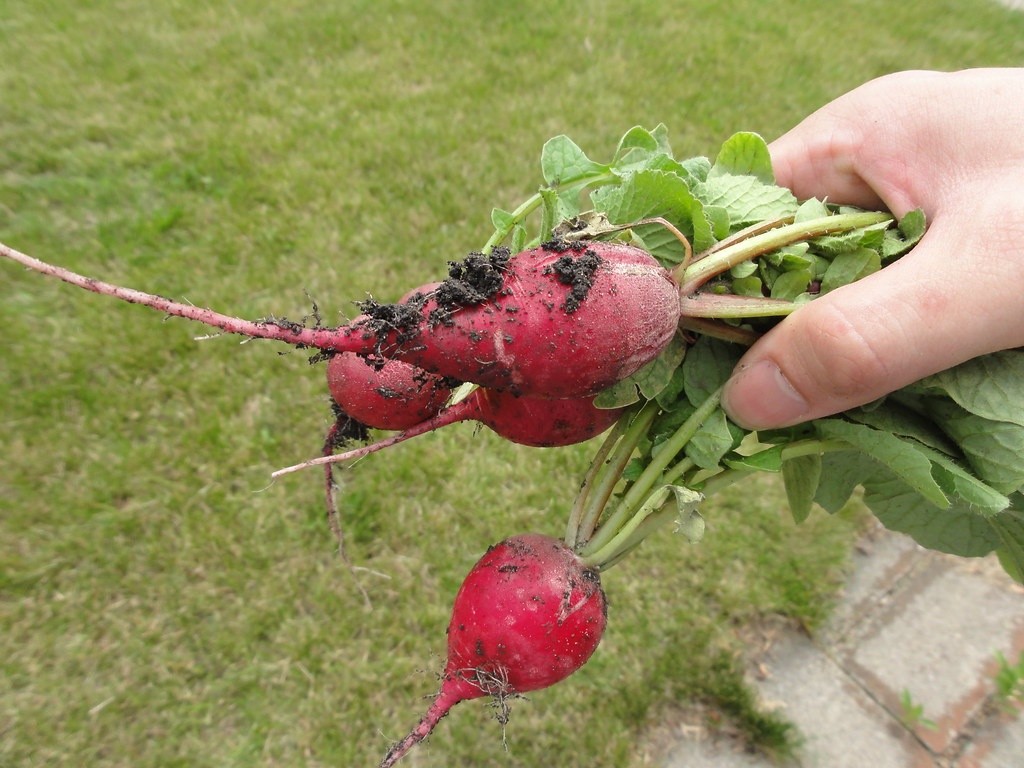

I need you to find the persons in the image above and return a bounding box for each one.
[720,67,1024,434]
